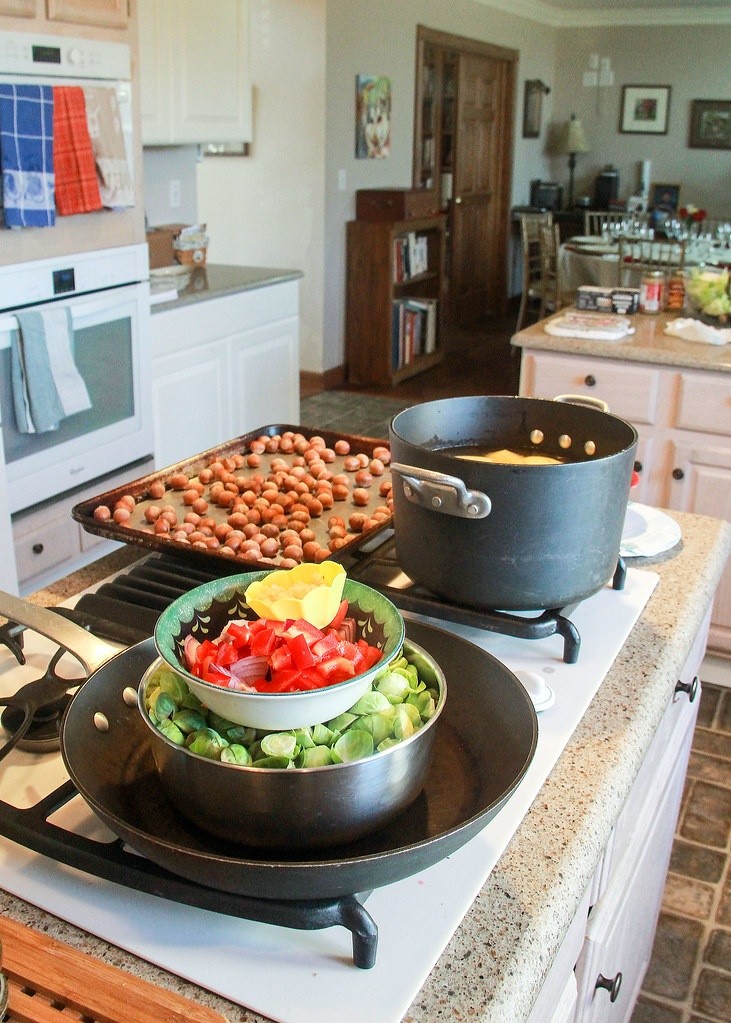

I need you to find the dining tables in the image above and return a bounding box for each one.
[555,232,730,308]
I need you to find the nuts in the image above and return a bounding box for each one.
[92,432,393,567]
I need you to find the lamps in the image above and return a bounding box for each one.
[553,109,591,209]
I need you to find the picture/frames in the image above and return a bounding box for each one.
[617,83,674,136]
[522,78,544,138]
[686,99,730,150]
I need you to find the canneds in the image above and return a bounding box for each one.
[638,271,666,315]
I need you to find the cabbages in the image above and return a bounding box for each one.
[145,647,436,770]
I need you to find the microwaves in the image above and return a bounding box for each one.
[1,31,134,179]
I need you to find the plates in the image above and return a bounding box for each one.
[571,236,602,244]
[620,502,682,557]
[576,244,602,252]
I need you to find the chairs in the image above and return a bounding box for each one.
[509,212,554,353]
[616,232,684,286]
[534,220,578,322]
[585,210,635,240]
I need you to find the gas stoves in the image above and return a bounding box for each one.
[3,523,660,1023]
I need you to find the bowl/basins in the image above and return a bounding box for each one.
[149,265,193,291]
[139,638,449,852]
[154,570,406,731]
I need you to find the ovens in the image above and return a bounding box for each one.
[0,280,155,517]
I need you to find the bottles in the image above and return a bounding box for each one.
[664,270,688,314]
[639,269,667,315]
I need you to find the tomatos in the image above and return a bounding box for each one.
[183,599,383,693]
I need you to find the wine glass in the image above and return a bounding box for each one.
[602,211,731,271]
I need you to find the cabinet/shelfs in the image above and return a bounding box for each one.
[509,350,729,688]
[534,595,730,1022]
[421,36,507,334]
[12,453,156,593]
[343,215,452,384]
[139,0,254,148]
[155,264,304,470]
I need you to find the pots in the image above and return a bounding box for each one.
[0,590,539,900]
[388,393,638,611]
